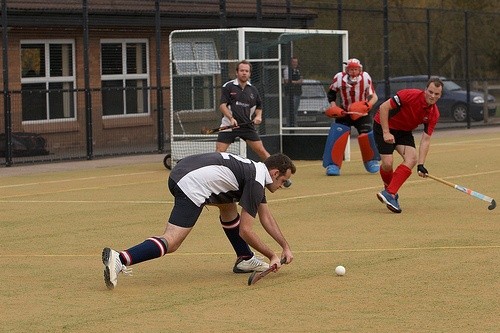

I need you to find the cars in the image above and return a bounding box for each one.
[297,79,335,125]
[372,74,497,123]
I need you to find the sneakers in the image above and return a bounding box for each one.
[102,247,132,290]
[376,190,402,213]
[233,252,271,273]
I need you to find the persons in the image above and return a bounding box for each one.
[101,153,296,289]
[284,56,303,127]
[216,60,291,187]
[322,59,380,175]
[373,78,444,213]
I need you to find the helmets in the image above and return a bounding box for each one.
[345,58,362,77]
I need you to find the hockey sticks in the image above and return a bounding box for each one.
[424,173,496,210]
[341,110,368,116]
[248,257,287,286]
[201,120,254,133]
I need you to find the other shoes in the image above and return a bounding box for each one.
[284,180,291,187]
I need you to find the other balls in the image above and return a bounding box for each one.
[335,265,346,276]
